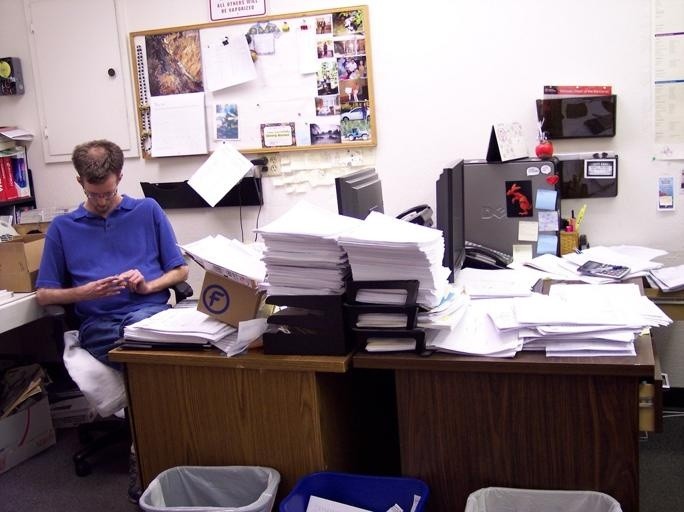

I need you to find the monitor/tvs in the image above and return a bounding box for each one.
[335,168,386,220]
[436,158,466,283]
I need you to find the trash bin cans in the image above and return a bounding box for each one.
[137,466,283,512]
[278,469,431,512]
[463,486,622,512]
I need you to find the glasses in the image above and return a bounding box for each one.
[82,181,119,199]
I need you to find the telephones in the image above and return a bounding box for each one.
[393,204,435,228]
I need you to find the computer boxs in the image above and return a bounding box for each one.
[462,156,562,256]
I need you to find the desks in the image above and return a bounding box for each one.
[109,287,356,510]
[353,278,655,511]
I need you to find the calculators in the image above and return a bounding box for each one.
[576,260,630,280]
[463,240,513,270]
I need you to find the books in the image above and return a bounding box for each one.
[0,135,31,202]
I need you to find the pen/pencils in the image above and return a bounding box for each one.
[588,265,628,274]
[564,204,588,232]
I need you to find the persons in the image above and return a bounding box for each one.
[316,15,369,124]
[35,140,189,505]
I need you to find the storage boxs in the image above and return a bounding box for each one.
[0,233,46,293]
[0,390,56,475]
[197,269,275,328]
